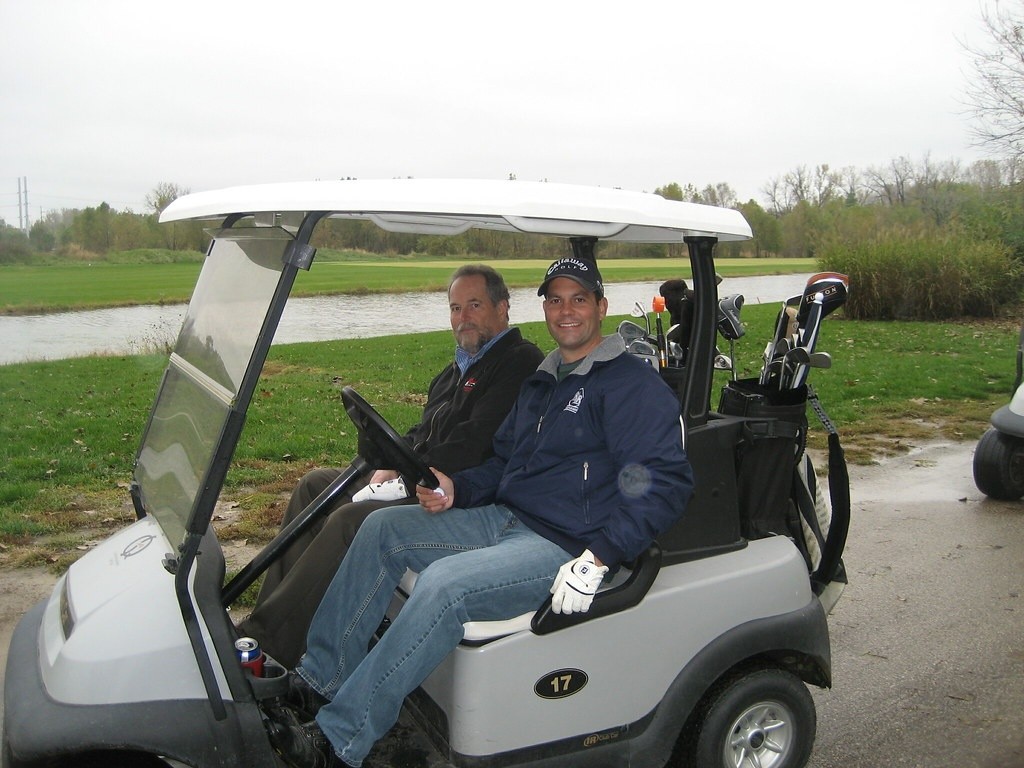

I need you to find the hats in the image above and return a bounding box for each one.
[538,258,605,300]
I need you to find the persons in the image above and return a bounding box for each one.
[264,255,691,768]
[234,263,544,666]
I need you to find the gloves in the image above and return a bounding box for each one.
[549,546,611,615]
[351,475,408,503]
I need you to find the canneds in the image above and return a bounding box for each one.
[233,637,260,663]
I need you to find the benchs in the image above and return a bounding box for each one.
[395,414,688,647]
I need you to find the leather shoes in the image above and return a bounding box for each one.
[264,674,325,723]
[264,718,337,768]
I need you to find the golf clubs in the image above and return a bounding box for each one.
[759,292,849,392]
[618,268,747,382]
